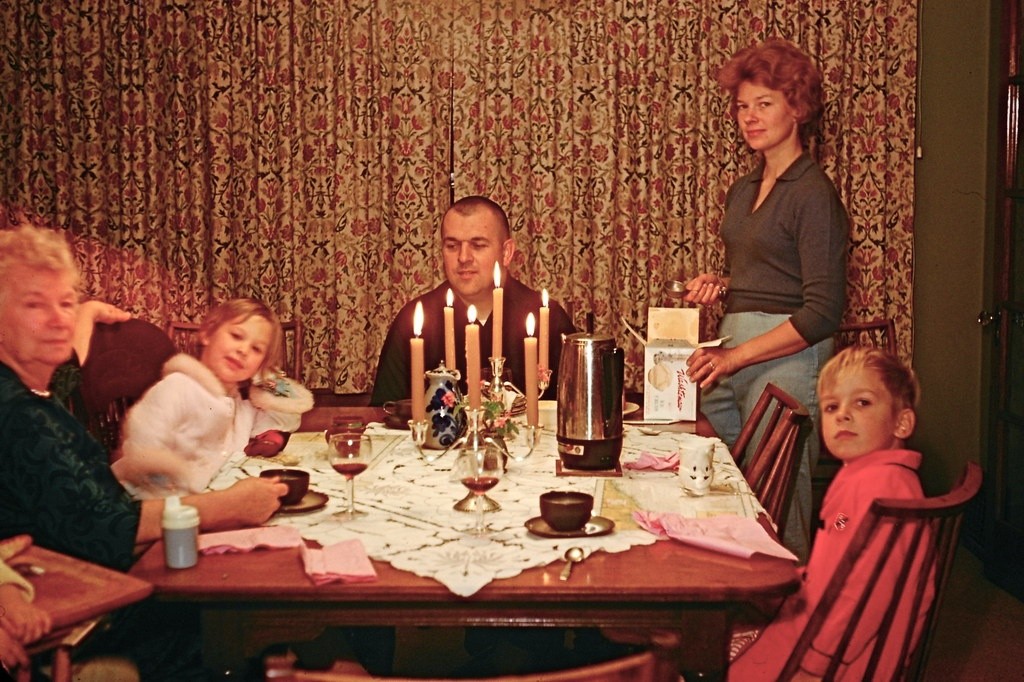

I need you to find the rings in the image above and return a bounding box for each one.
[709,361,715,369]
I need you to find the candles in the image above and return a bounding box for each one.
[410,301,424,425]
[443,288,455,370]
[538,290,549,381]
[524,313,538,426]
[492,262,503,359]
[465,305,481,409]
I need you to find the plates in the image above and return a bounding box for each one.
[384,416,410,430]
[524,516,615,539]
[277,490,330,514]
[622,402,640,415]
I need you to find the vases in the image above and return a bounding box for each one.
[464,429,509,475]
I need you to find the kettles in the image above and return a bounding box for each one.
[555,313,625,472]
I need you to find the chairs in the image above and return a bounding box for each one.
[264,314,983,682]
[167,316,303,386]
[0,542,154,682]
[63,316,175,454]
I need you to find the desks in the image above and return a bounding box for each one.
[126,408,800,682]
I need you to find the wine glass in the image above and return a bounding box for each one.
[327,433,371,521]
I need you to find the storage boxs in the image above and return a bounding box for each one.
[619,307,734,421]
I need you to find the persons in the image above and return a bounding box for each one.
[0,223,289,682]
[0,558,52,669]
[110,298,313,500]
[243,196,579,680]
[683,37,850,569]
[655,348,939,682]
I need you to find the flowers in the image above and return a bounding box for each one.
[442,377,519,439]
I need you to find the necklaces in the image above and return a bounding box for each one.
[31,388,50,396]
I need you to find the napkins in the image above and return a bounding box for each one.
[620,449,680,471]
[631,506,799,561]
[300,537,379,586]
[196,526,301,556]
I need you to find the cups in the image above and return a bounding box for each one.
[259,468,310,507]
[160,506,200,569]
[382,399,412,418]
[677,442,716,497]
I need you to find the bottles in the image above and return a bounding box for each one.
[486,356,506,397]
[325,416,366,443]
